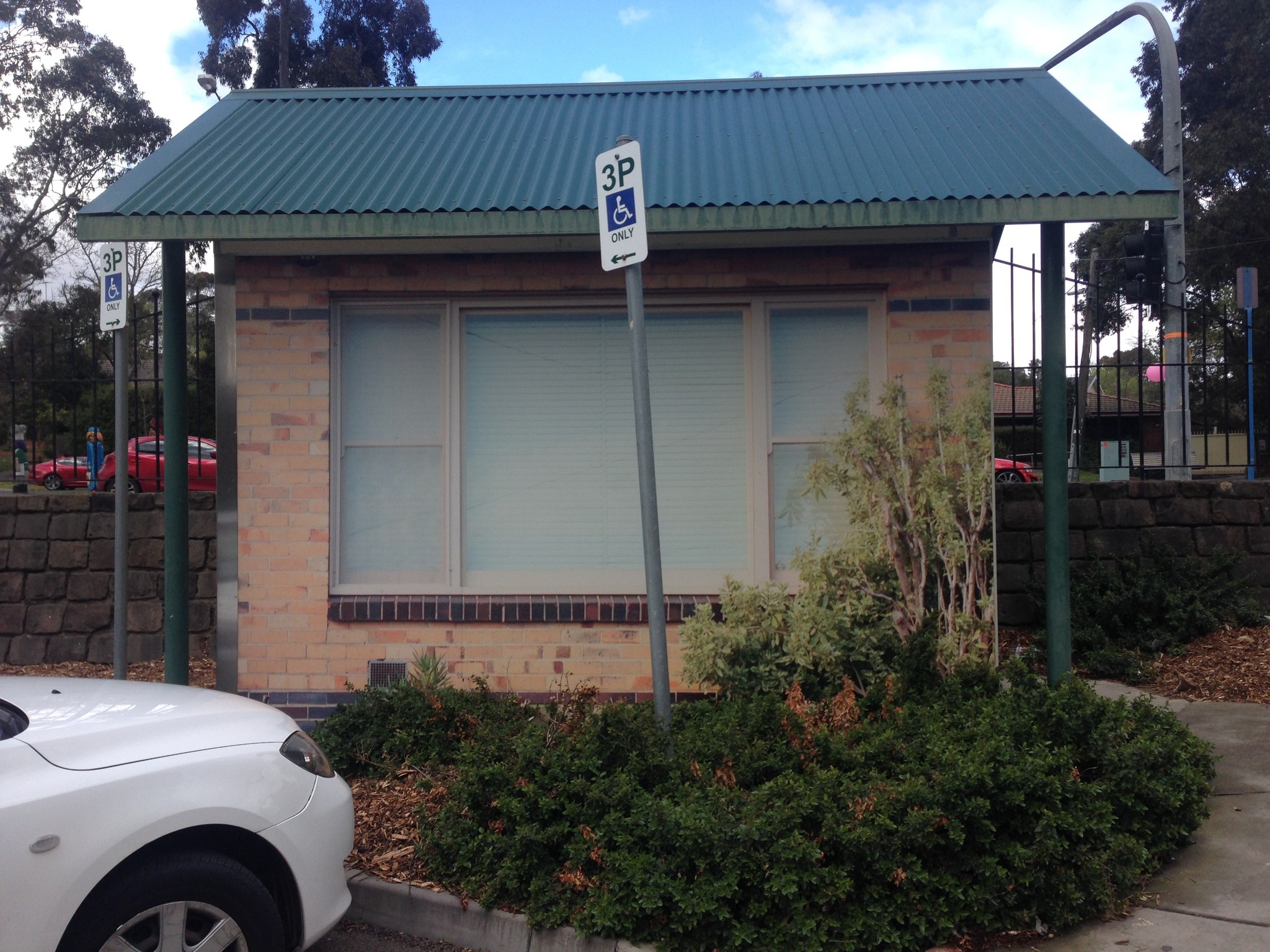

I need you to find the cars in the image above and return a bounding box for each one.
[28,456,88,491]
[0,673,355,952]
[95,436,216,493]
[994,458,1039,485]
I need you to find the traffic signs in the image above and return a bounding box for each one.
[595,140,649,271]
[100,242,126,331]
